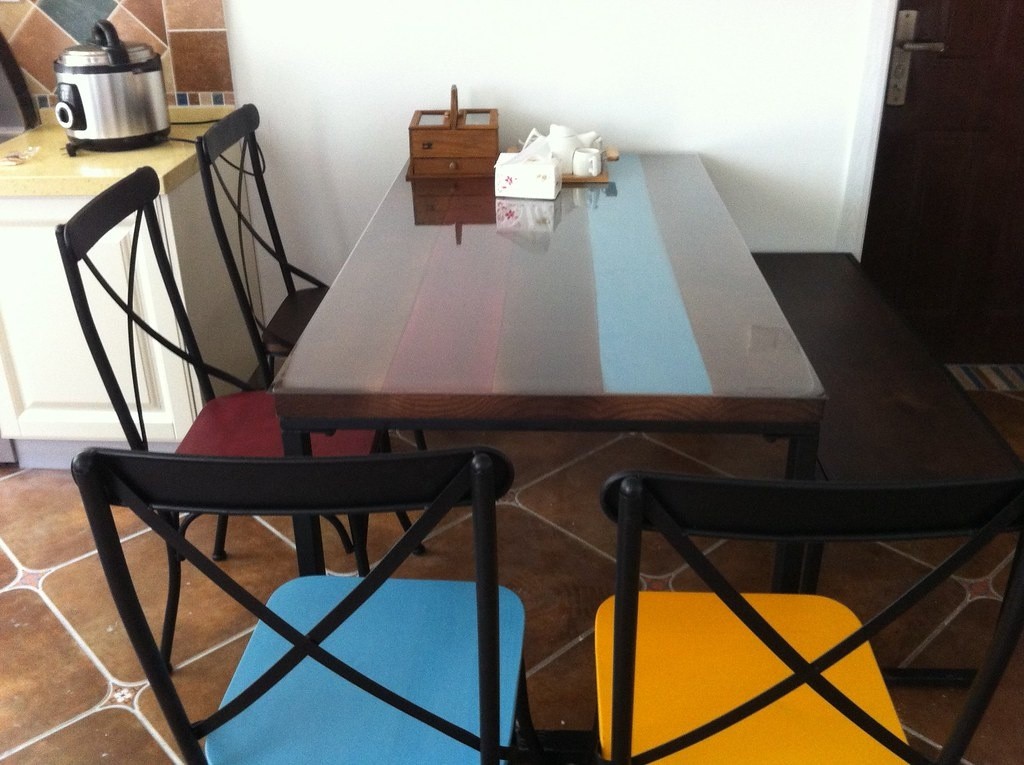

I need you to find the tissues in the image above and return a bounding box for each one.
[493,136,562,200]
[495,198,562,255]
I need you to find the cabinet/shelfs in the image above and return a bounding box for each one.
[0,105,270,474]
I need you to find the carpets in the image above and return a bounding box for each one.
[942,360,1024,479]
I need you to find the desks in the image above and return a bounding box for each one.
[264,149,830,592]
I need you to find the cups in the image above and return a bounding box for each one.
[573,148,601,177]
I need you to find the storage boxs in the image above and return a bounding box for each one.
[408,109,499,176]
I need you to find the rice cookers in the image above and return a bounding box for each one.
[54,19,170,156]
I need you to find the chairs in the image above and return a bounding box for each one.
[55,101,1024,765]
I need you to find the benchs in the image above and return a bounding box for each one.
[748,251,1024,696]
